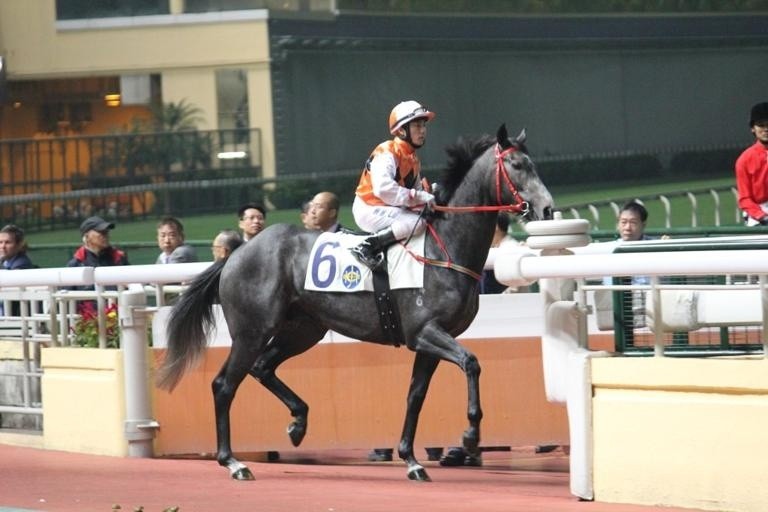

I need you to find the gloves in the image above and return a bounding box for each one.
[413,190,438,213]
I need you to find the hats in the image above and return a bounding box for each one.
[78,216,116,236]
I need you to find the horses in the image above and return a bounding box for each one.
[152,120,557,485]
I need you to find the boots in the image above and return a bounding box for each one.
[348,223,397,277]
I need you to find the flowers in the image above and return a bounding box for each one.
[72,301,120,349]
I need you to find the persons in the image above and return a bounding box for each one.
[602,201,670,286]
[1,224,45,315]
[154,217,196,264]
[60,216,130,315]
[299,191,353,234]
[238,203,266,242]
[480,211,528,293]
[352,100,436,268]
[211,229,244,262]
[735,102,768,226]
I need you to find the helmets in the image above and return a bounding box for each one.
[749,101,768,125]
[386,99,436,136]
